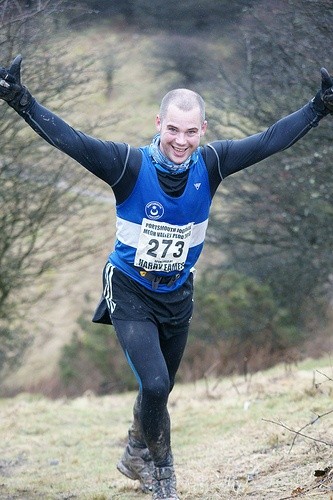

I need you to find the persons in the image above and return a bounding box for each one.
[0,54,333,499]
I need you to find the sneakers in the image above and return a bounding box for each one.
[117,446,155,494]
[151,468,177,499]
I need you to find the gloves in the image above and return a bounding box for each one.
[314,67,333,115]
[0,54,36,116]
